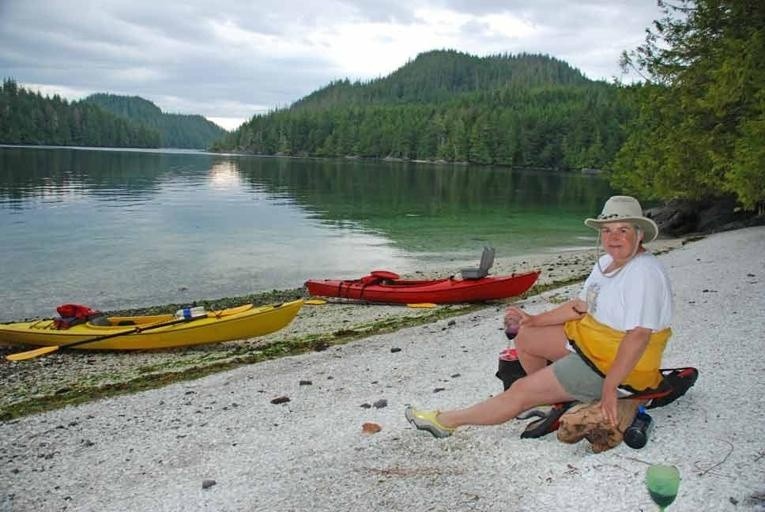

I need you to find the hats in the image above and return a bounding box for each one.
[584,196,659,244]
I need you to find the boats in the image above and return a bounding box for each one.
[1,297,303,353]
[306,268,541,303]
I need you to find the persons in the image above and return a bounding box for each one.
[403,195,675,439]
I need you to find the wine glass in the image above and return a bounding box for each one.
[646,464,680,512]
[498,316,521,361]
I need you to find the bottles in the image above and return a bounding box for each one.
[623,412,655,449]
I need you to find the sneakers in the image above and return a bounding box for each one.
[405,407,454,438]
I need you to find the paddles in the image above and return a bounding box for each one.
[6,303,255,361]
[304,299,436,309]
